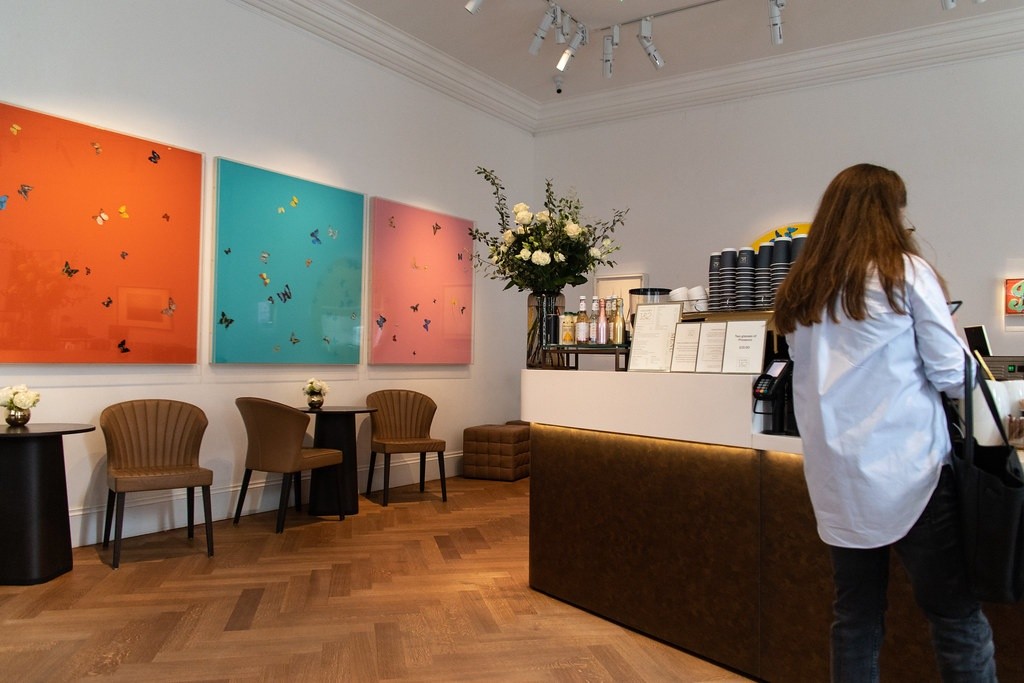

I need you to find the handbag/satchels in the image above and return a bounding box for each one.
[941,352,1024,603]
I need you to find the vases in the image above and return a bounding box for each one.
[308,393,324,407]
[6,406,31,425]
[528,290,566,368]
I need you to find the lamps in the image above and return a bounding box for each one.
[463,0,786,78]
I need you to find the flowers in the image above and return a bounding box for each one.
[0,385,40,408]
[304,377,328,396]
[461,167,627,291]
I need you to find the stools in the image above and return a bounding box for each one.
[462,421,533,481]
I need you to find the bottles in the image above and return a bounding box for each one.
[558,295,625,346]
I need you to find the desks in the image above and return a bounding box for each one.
[0,421,95,588]
[296,404,378,515]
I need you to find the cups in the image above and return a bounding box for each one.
[668,233,808,313]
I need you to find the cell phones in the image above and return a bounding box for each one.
[948,299,963,315]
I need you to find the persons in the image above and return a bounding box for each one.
[775,164,997,683]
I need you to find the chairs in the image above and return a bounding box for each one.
[102,398,214,568]
[233,396,346,534]
[367,388,448,506]
